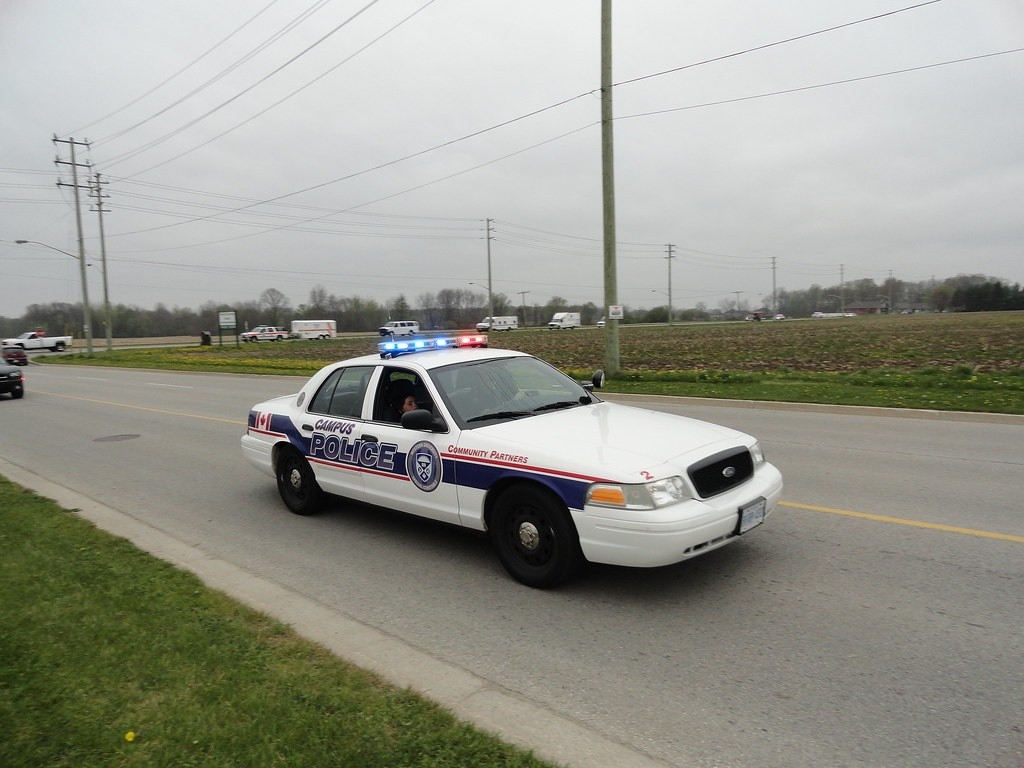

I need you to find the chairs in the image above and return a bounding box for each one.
[454,367,479,390]
[382,378,415,424]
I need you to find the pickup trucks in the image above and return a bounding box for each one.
[241,326,288,342]
[0,332,73,352]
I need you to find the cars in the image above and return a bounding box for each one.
[745,313,762,322]
[775,314,786,320]
[597,315,605,328]
[0,345,27,366]
[240,332,784,593]
[0,354,24,399]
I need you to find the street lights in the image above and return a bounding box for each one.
[468,281,493,333]
[15,240,94,360]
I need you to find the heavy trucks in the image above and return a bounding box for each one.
[274,319,337,340]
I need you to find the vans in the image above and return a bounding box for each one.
[548,312,581,330]
[476,316,519,333]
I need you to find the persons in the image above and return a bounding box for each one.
[397,394,418,413]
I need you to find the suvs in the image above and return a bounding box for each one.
[378,321,420,337]
